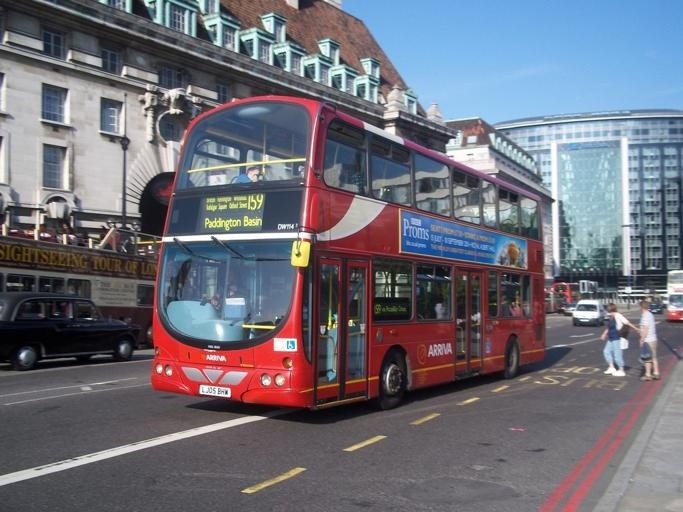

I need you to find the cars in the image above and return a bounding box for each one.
[557,300,611,327]
[644,296,663,314]
[0,290,139,371]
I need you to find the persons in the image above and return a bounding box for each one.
[231,167,260,184]
[599,301,661,381]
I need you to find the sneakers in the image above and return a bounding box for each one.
[640,373,660,380]
[604,368,626,376]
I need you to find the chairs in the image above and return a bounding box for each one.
[202,162,359,192]
[222,297,247,319]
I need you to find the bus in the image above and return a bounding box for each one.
[144,96,550,414]
[551,283,580,315]
[665,269,682,321]
